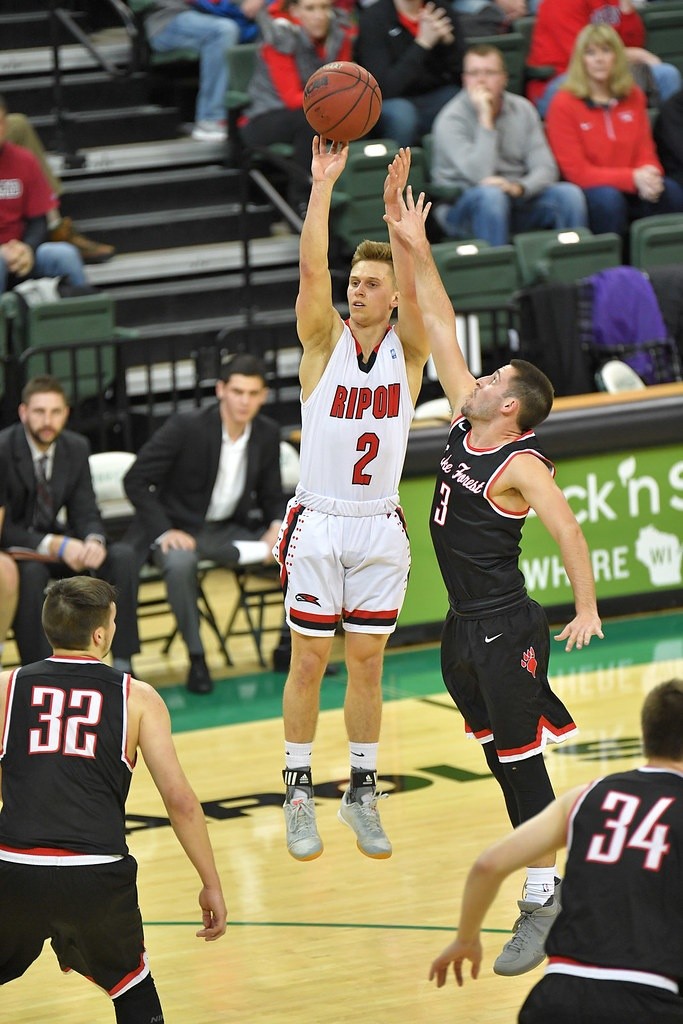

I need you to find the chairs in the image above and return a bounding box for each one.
[58,434,298,665]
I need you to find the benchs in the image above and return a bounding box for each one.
[1,0,683,401]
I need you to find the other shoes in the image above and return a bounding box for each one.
[51,215,115,263]
[273,650,337,675]
[187,654,212,694]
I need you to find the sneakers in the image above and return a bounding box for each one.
[283,796,323,862]
[338,791,393,860]
[495,876,564,977]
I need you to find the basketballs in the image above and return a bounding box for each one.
[302,60,382,142]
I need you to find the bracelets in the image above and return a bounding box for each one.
[57,536,71,558]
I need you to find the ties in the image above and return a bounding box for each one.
[35,455,55,534]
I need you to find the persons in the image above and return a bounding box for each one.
[0,374,139,681]
[0,0,683,303]
[0,575,227,1024]
[384,184,605,976]
[429,679,683,1024]
[121,355,340,694]
[269,132,429,858]
